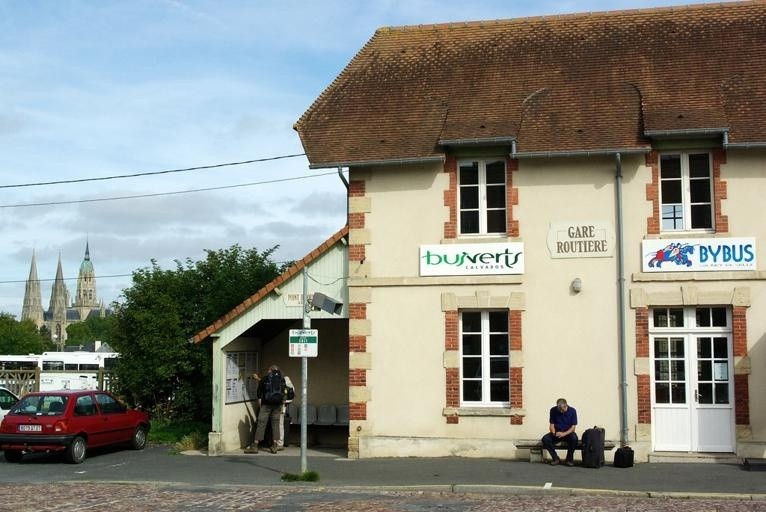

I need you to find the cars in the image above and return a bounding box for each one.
[0,386,150,463]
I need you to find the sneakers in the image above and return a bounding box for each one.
[565,460,574,467]
[270,441,290,454]
[244,443,258,453]
[551,459,560,466]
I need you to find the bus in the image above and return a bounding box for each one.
[0,352,119,403]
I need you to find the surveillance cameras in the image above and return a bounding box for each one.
[312,292,343,316]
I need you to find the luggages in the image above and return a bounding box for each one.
[581,425,605,468]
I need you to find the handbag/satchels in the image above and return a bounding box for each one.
[287,387,295,400]
[614,446,634,468]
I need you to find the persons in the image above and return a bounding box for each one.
[541,399,578,467]
[252,373,295,448]
[243,364,286,453]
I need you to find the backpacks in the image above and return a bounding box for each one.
[263,371,285,404]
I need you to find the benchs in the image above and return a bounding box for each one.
[516,440,615,466]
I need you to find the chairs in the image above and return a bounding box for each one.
[285,403,348,453]
[49,401,63,412]
